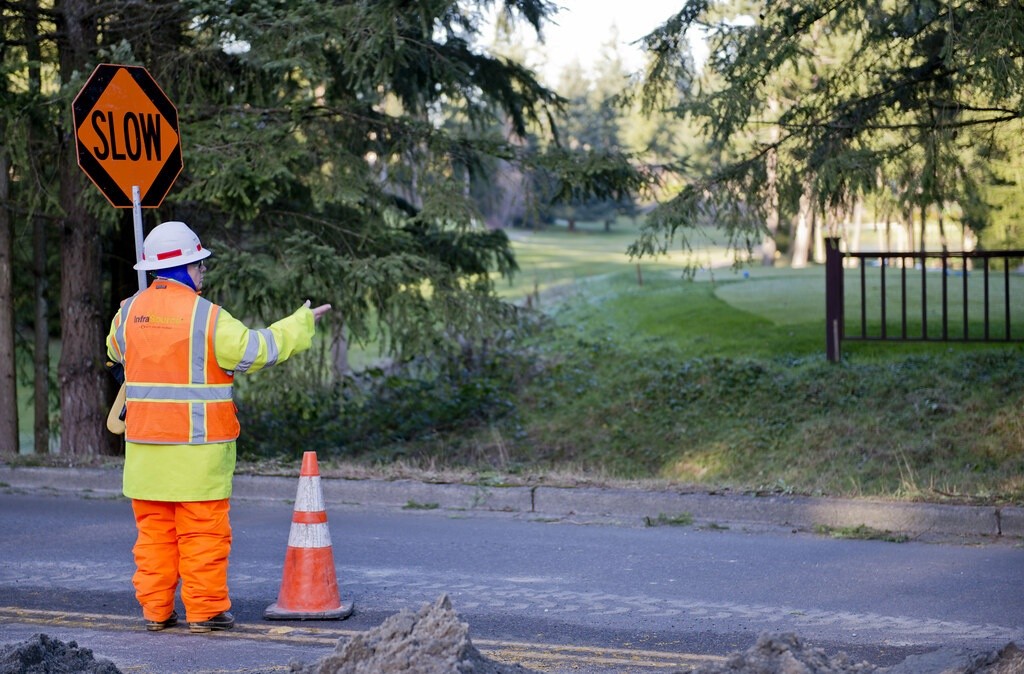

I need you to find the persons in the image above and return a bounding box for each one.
[106,221,331,632]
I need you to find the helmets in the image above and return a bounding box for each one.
[133,221,211,271]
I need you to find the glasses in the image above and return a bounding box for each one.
[190,261,204,269]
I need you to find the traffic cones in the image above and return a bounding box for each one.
[262,451,355,620]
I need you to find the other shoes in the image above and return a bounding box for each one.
[145,611,178,631]
[189,613,235,634]
